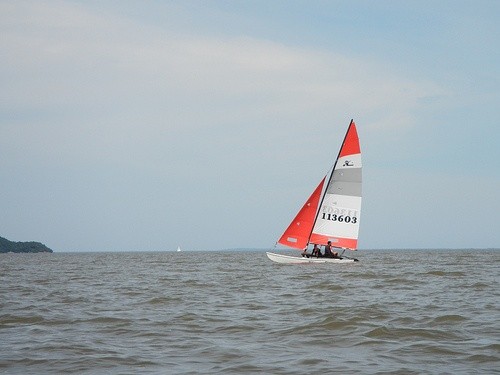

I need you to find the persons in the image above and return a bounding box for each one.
[324,240,338,258]
[312,243,323,257]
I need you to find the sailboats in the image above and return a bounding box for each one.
[265,117,363,263]
